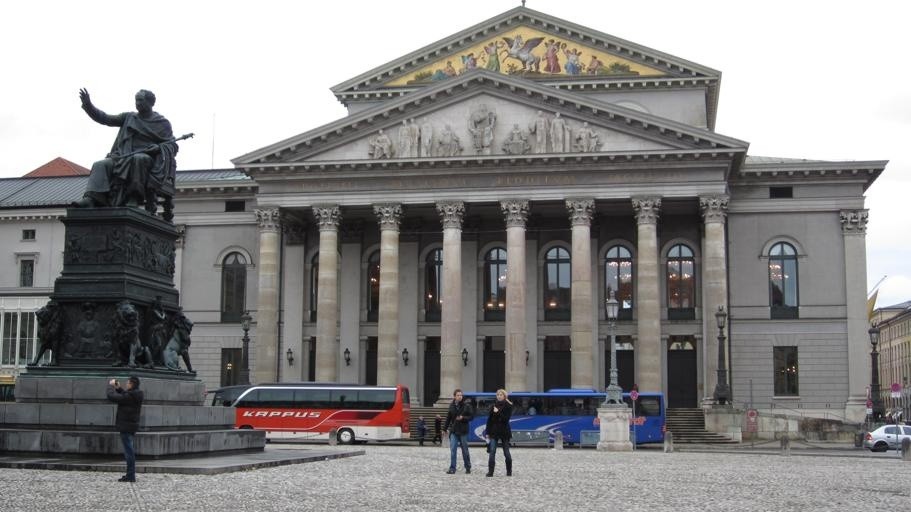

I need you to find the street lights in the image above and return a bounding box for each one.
[234,307,252,386]
[864,318,889,423]
[593,287,635,454]
[710,304,732,407]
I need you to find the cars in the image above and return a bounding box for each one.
[859,423,911,453]
[876,406,903,423]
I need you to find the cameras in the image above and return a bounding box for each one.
[115,382,119,386]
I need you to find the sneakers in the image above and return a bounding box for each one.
[446,471,455,474]
[466,470,470,474]
[118,476,136,482]
[507,470,512,476]
[485,472,493,477]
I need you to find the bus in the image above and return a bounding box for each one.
[1,374,15,404]
[460,386,668,448]
[203,381,414,445]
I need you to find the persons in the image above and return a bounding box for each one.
[415,415,430,445]
[367,102,599,159]
[542,39,561,73]
[486,388,513,477]
[432,414,442,444]
[443,388,474,474]
[106,375,144,483]
[480,41,505,71]
[71,87,180,208]
[562,47,582,73]
[586,55,602,74]
[464,51,484,69]
[444,61,456,73]
